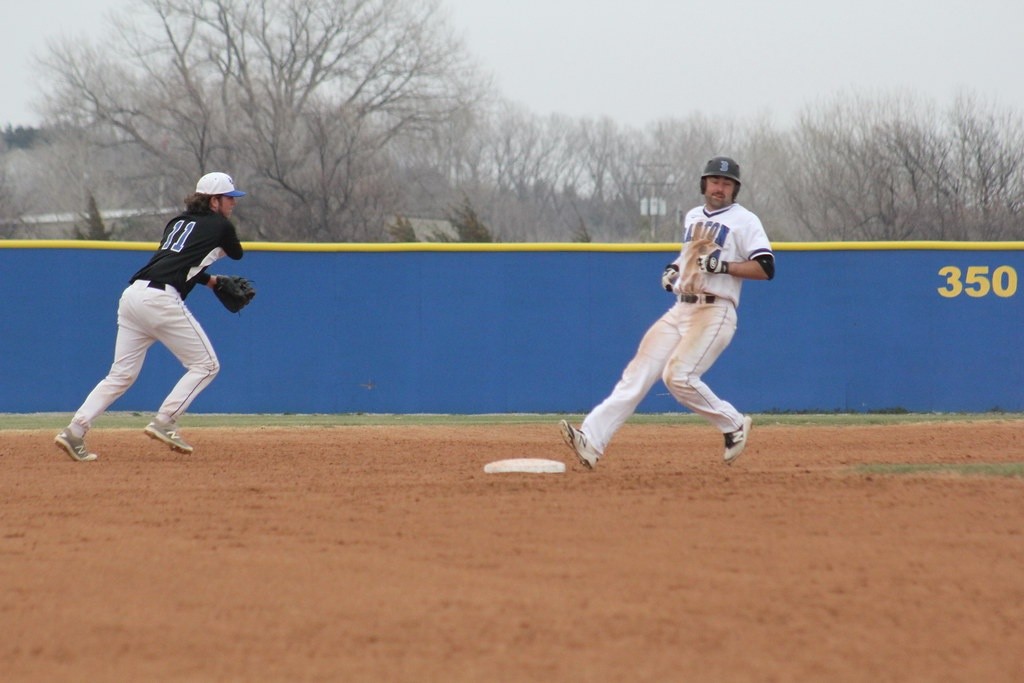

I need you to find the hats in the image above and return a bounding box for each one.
[195,172,247,197]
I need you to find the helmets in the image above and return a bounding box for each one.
[700,156,742,198]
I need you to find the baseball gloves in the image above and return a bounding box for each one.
[213,275,256,313]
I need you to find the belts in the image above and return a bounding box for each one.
[681,294,715,304]
[147,280,166,292]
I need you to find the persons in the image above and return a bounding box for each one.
[560,156,775,470]
[54,172,255,461]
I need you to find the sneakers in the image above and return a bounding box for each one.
[558,419,598,468]
[144,418,195,455]
[723,416,753,462]
[52,427,97,462]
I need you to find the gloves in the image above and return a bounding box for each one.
[697,254,729,275]
[660,264,678,292]
